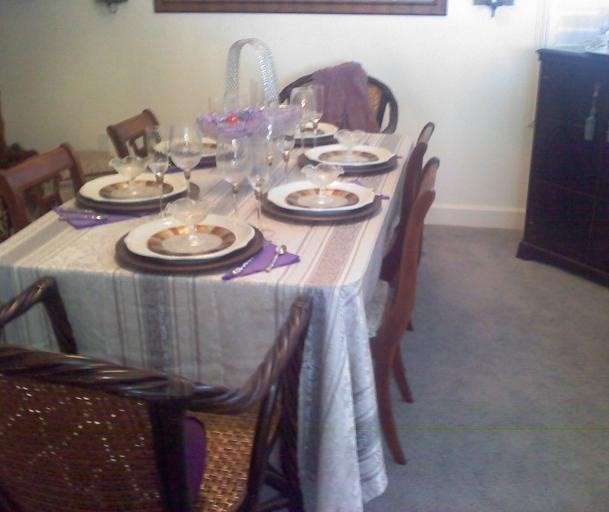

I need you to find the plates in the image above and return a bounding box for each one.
[79,173,188,204]
[76,181,201,213]
[260,194,382,223]
[298,155,396,175]
[266,180,375,215]
[116,225,264,270]
[124,215,254,260]
[305,146,393,165]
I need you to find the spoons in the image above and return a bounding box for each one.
[265,245,288,271]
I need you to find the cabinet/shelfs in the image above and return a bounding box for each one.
[515,48,609,289]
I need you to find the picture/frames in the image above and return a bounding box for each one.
[154,1,447,14]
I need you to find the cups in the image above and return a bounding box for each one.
[109,156,151,199]
[299,163,346,205]
[166,198,209,244]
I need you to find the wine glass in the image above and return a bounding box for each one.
[145,126,170,218]
[210,82,322,207]
[173,124,204,198]
